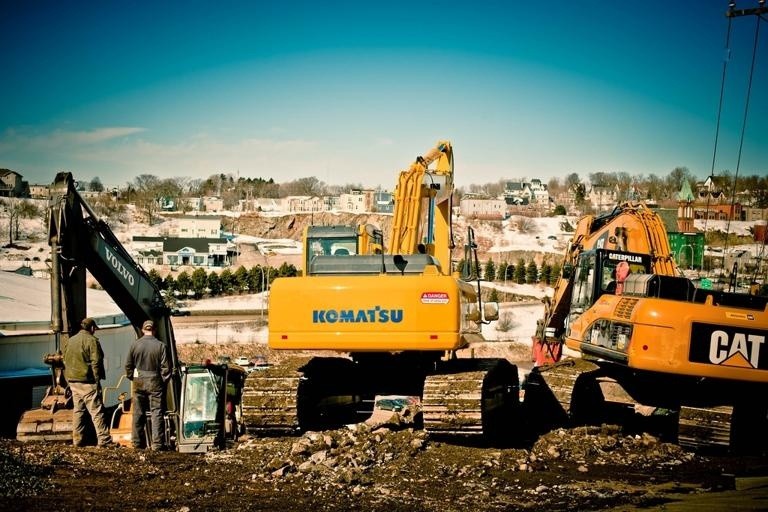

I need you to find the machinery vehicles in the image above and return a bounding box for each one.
[17,169,249,455]
[519,198,767,454]
[236,138,519,438]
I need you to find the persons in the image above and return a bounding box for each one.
[64,318,121,449]
[125,320,172,451]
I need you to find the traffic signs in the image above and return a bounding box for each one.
[702,279,711,288]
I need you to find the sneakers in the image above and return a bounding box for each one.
[132,439,170,452]
[96,441,120,449]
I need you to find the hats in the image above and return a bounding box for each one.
[81,318,100,330]
[142,320,155,331]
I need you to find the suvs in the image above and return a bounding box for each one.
[216,355,231,365]
[249,354,268,364]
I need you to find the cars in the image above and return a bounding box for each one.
[235,356,249,366]
